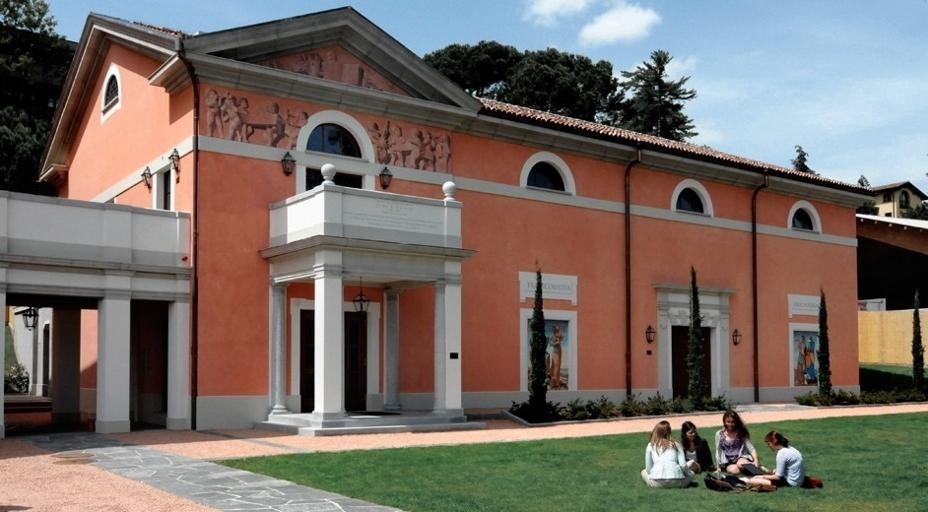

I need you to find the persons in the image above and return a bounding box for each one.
[714,409,769,475]
[369,120,451,172]
[746,431,805,490]
[798,336,817,385]
[549,325,561,387]
[205,89,308,150]
[641,420,697,488]
[680,421,717,473]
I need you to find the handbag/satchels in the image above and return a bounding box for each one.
[704,473,777,492]
